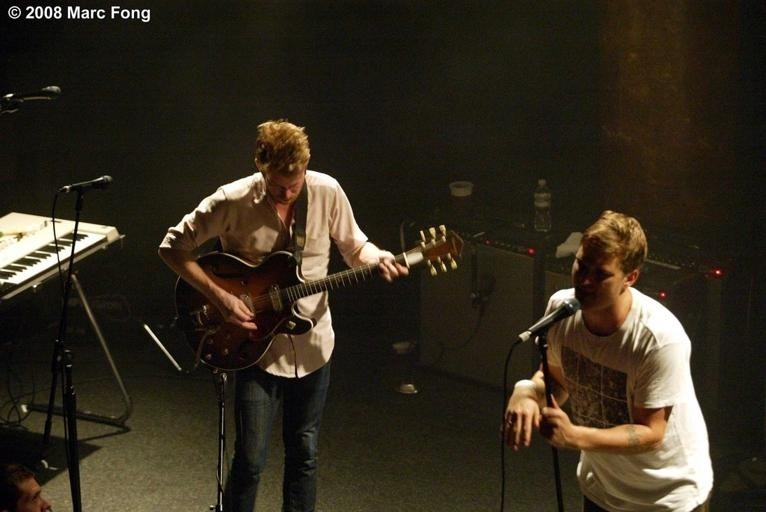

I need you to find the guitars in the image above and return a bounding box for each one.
[174,225,464,372]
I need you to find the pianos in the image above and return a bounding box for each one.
[0,219,126,302]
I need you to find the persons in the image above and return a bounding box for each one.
[0,463,53,512]
[500,209,716,512]
[157,120,410,511]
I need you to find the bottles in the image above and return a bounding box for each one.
[534,179,554,233]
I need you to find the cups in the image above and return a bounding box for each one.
[449,181,473,214]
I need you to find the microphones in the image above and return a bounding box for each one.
[0,84,65,107]
[518,298,580,343]
[60,174,115,197]
